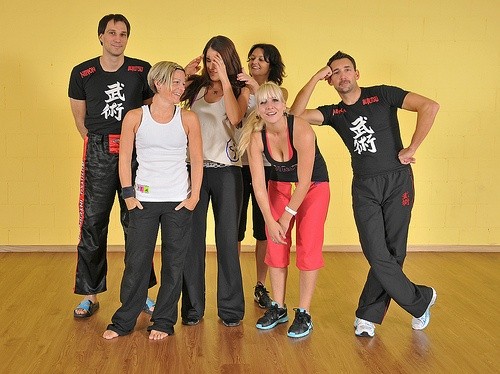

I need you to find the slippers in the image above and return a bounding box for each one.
[142,296,155,315]
[73,298,100,318]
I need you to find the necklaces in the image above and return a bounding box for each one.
[211,85,221,94]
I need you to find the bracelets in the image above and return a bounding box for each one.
[119,186,137,200]
[284,206,298,216]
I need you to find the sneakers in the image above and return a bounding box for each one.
[252,285,274,309]
[353,316,377,338]
[255,302,289,330]
[286,308,314,338]
[412,286,437,330]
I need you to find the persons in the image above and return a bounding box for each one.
[237,43,289,309]
[102,61,204,341]
[237,82,330,337]
[181,35,250,326]
[68,13,156,319]
[288,50,438,337]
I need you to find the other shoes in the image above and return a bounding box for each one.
[220,318,242,327]
[182,317,200,325]
